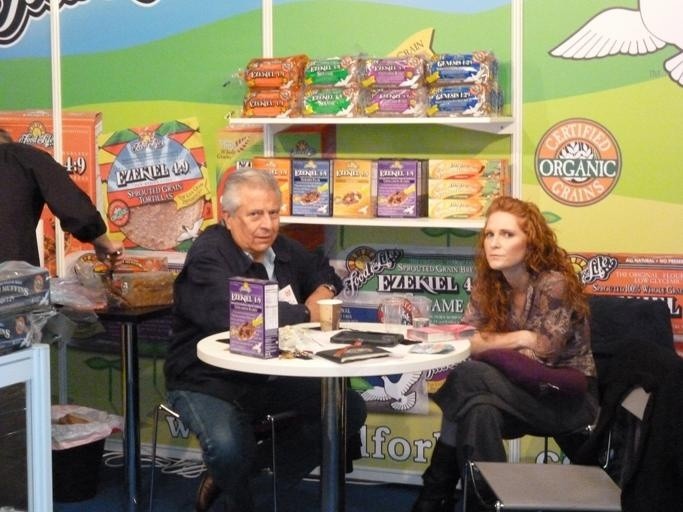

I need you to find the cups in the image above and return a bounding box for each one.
[315,298,342,331]
[382,299,430,332]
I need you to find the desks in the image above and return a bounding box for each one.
[46,303,178,511]
[196,322,472,511]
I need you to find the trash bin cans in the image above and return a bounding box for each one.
[48,405,111,503]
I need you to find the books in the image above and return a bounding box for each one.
[330,329,403,348]
[313,346,393,364]
[402,324,478,343]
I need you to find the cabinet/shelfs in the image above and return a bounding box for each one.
[229,114,512,236]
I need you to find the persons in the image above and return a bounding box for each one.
[166,165,368,511]
[410,196,603,512]
[0,127,124,270]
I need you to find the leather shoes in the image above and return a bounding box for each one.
[194,473,218,512]
[223,484,249,512]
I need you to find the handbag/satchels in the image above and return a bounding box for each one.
[475,349,588,402]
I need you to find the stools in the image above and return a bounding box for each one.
[151,401,273,512]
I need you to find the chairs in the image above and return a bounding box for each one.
[589,295,682,512]
[462,379,657,510]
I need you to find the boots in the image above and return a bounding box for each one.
[411,439,459,512]
